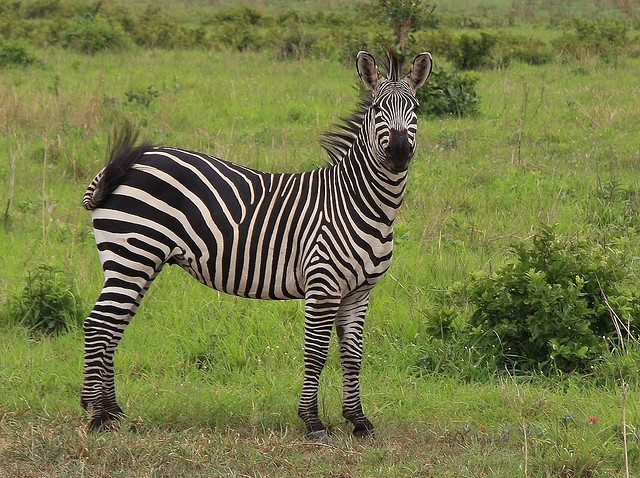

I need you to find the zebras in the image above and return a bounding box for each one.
[82,32,432,441]
